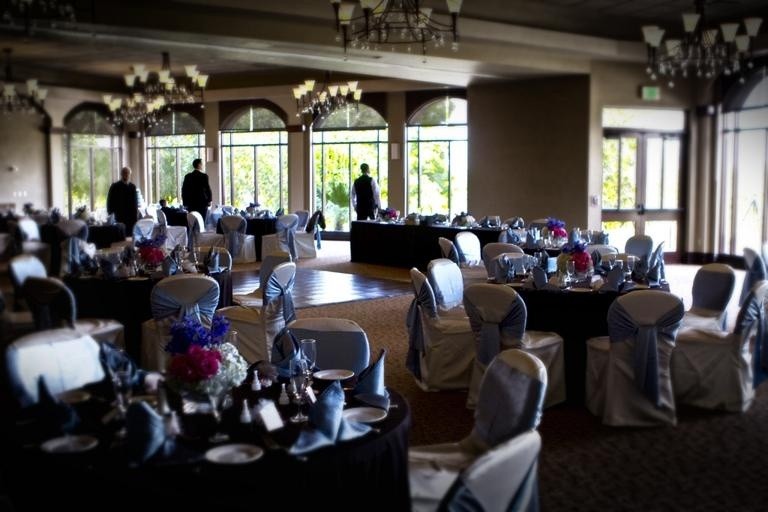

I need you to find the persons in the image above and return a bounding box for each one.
[106,166,138,237]
[159,199,191,252]
[350,163,382,220]
[181,159,212,228]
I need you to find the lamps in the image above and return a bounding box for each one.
[290,70,365,127]
[641,2,763,90]
[101,52,208,134]
[1,74,47,111]
[332,1,461,55]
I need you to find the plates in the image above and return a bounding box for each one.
[314,369,355,381]
[41,435,99,454]
[342,407,388,424]
[205,444,264,463]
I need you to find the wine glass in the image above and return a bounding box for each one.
[205,375,229,443]
[614,259,624,270]
[521,252,542,282]
[565,260,576,283]
[224,331,240,348]
[626,255,635,284]
[301,339,317,379]
[289,359,310,424]
